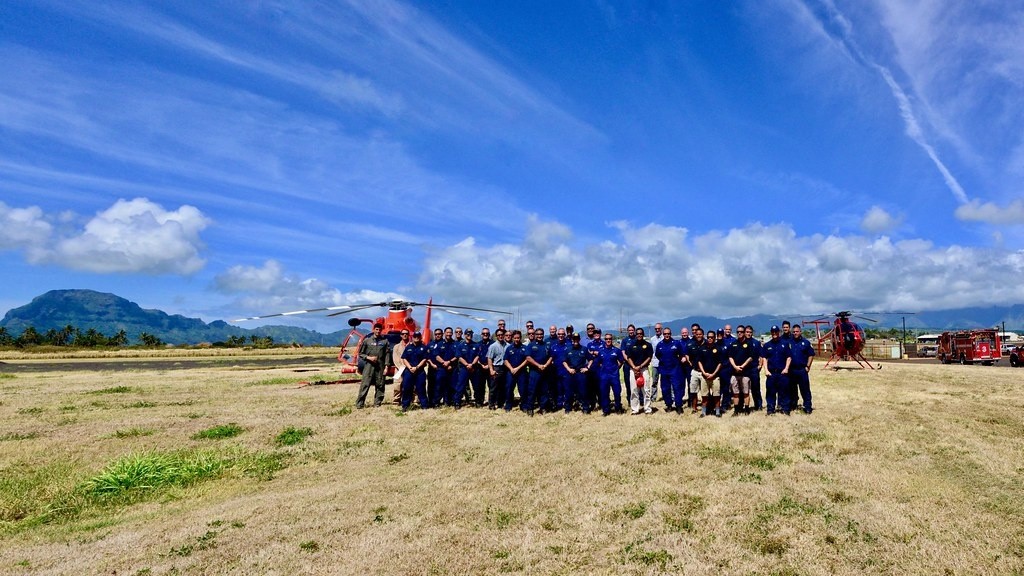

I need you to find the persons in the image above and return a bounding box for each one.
[393,329,420,406]
[425,319,815,417]
[356,323,390,409]
[401,331,431,412]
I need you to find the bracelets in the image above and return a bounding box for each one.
[450,360,452,363]
[807,366,810,369]
[587,367,589,369]
[416,365,419,369]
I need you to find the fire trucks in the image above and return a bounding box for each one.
[938,326,1001,366]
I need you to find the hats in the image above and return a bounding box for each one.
[572,334,580,340]
[636,374,644,387]
[464,328,473,335]
[771,325,779,332]
[592,328,601,333]
[717,328,724,335]
[566,325,573,332]
[413,332,421,337]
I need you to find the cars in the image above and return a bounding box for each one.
[917,348,936,358]
[1010,343,1024,367]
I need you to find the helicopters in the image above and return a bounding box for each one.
[768,310,916,371]
[231,300,513,378]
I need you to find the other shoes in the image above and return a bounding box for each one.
[677,407,683,414]
[700,414,706,418]
[716,414,721,418]
[732,411,739,417]
[667,406,672,413]
[691,410,696,414]
[746,409,750,415]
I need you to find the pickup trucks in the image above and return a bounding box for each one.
[1001,343,1017,355]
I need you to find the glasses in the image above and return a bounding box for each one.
[496,334,504,337]
[605,338,612,340]
[707,337,713,339]
[737,331,744,334]
[482,333,489,335]
[528,333,535,336]
[401,333,409,335]
[527,325,533,328]
[435,332,442,336]
